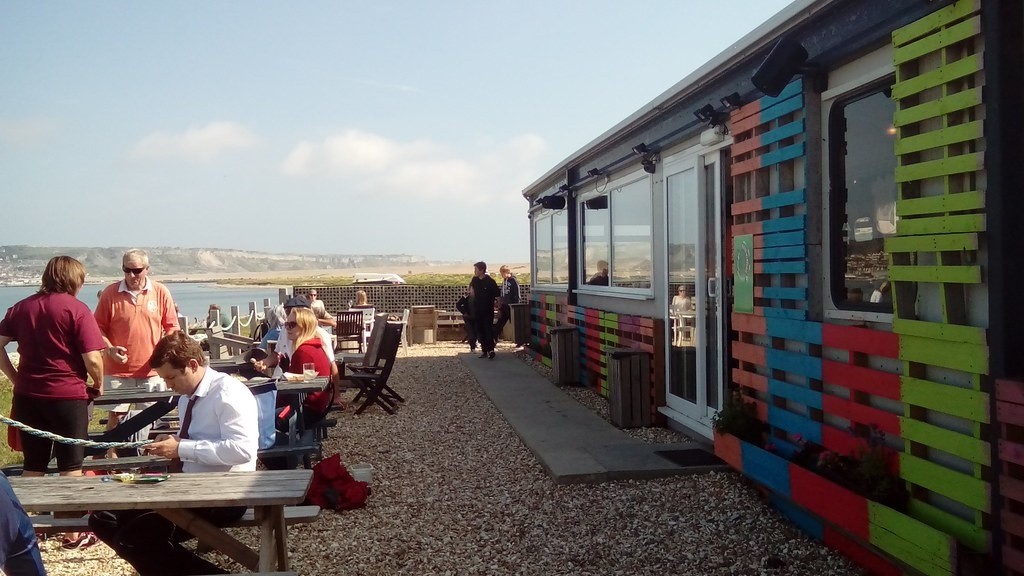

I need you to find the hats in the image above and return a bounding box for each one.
[283,295,311,308]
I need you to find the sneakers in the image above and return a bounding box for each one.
[60,533,103,552]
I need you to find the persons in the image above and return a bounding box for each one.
[0,256,100,554]
[456,262,520,359]
[250,290,341,470]
[206,304,233,334]
[355,290,366,305]
[672,286,691,312]
[93,249,182,470]
[0,468,47,576]
[90,331,258,576]
[589,261,608,285]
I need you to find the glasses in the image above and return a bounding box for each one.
[310,294,317,296]
[123,263,145,275]
[679,289,686,292]
[284,321,297,329]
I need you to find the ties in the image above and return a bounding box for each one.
[167,396,200,474]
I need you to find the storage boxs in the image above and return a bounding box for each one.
[350,464,374,483]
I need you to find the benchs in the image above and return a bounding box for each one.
[46,442,320,474]
[87,419,338,440]
[437,309,501,326]
[30,504,320,534]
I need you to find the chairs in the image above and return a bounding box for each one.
[339,310,388,377]
[410,305,438,344]
[341,322,405,417]
[347,307,376,353]
[335,310,364,353]
[207,326,252,359]
[386,307,411,354]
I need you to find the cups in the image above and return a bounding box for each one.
[302,363,315,382]
[110,380,121,389]
[156,382,166,392]
[144,383,154,393]
[349,299,353,307]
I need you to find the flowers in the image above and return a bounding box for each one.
[765,433,849,478]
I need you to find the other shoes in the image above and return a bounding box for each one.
[488,352,496,359]
[478,352,487,358]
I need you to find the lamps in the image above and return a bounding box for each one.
[694,105,731,127]
[640,146,661,174]
[632,143,651,155]
[558,184,576,194]
[587,168,610,179]
[720,91,744,110]
[535,198,542,205]
[750,36,830,99]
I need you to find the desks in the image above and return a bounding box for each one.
[45,376,331,476]
[210,355,344,441]
[8,470,312,576]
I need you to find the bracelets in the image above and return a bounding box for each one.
[108,346,114,357]
